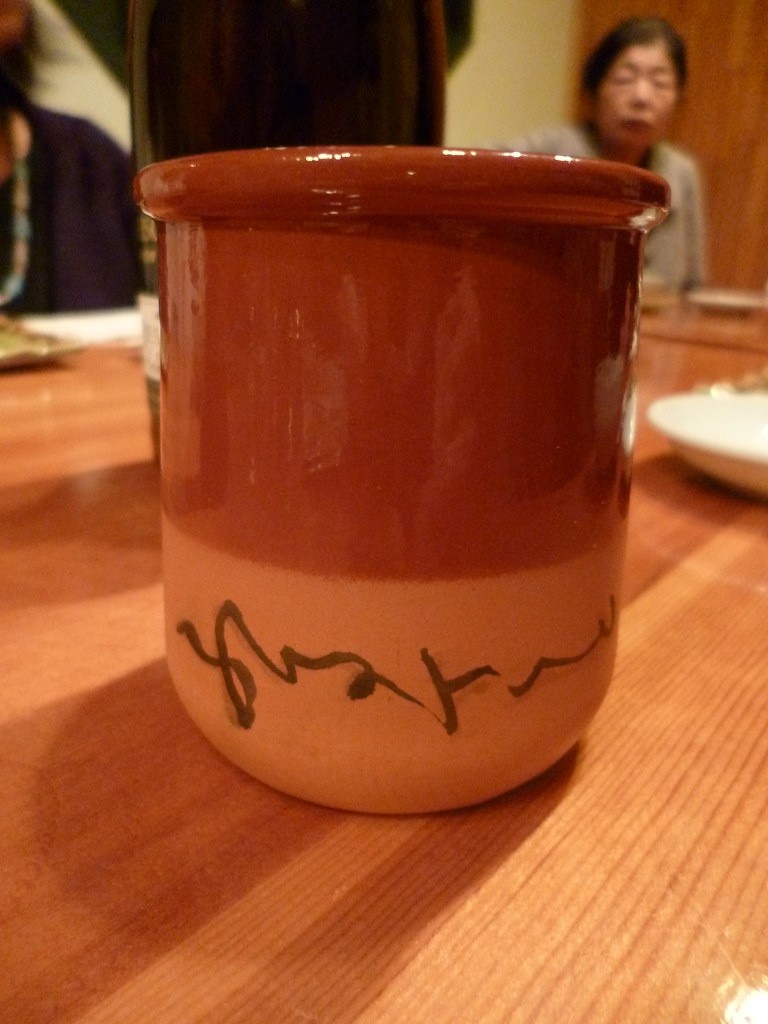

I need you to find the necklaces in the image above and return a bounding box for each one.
[0,111,33,314]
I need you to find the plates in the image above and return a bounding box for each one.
[687,287,766,313]
[0,329,91,368]
[647,394,767,498]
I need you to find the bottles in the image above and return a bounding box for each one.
[128,0,450,472]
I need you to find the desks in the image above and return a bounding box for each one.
[0,306,768,1024]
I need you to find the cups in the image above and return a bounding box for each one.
[133,145,671,815]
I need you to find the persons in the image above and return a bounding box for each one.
[0,0,140,322]
[495,15,708,298]
